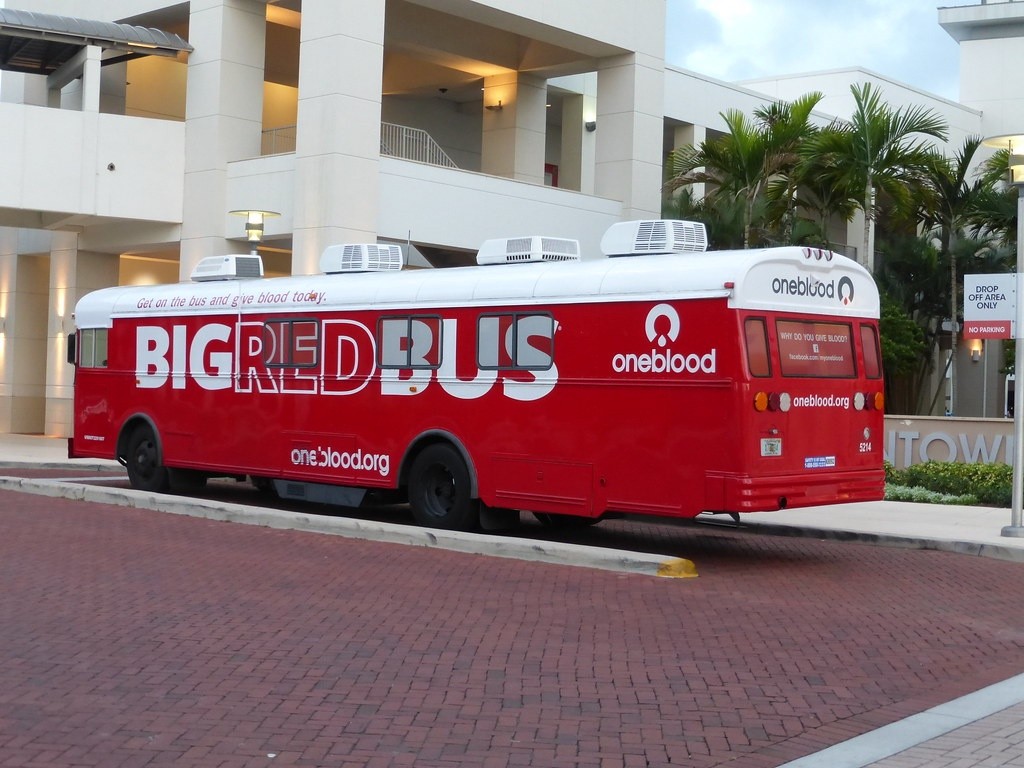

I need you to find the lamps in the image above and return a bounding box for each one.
[973,350,980,361]
[485,105,503,111]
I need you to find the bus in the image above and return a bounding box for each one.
[67,216,887,534]
